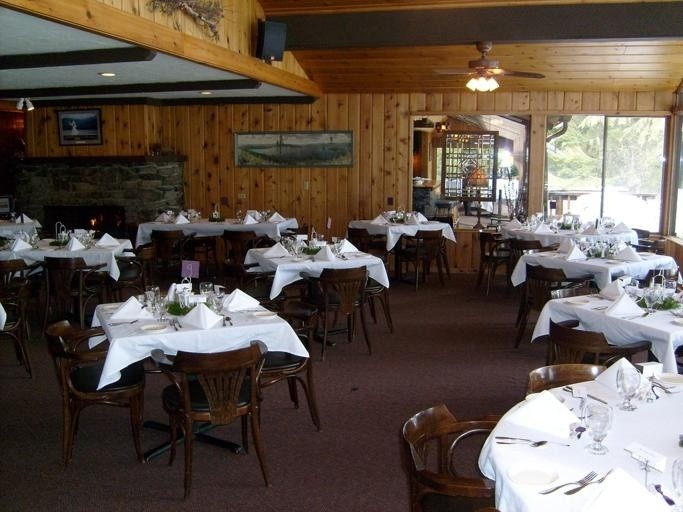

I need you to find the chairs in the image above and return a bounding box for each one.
[632,227,650,253]
[523,364,609,395]
[346,226,370,251]
[134,241,159,288]
[176,233,212,278]
[220,229,255,293]
[234,262,285,310]
[0,276,33,379]
[398,230,446,293]
[511,237,559,258]
[513,264,570,349]
[41,256,80,335]
[197,260,245,299]
[473,229,513,294]
[0,258,32,342]
[364,237,401,332]
[545,317,651,366]
[99,284,145,313]
[168,344,270,501]
[43,320,148,467]
[257,277,322,429]
[398,403,504,512]
[300,265,374,364]
[151,228,186,271]
[634,236,656,253]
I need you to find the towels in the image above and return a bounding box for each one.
[247,210,263,220]
[155,213,171,222]
[506,218,523,228]
[598,358,646,391]
[23,214,32,224]
[66,238,84,252]
[267,212,283,222]
[95,234,119,248]
[590,470,656,509]
[174,215,190,224]
[557,239,575,253]
[264,243,292,258]
[371,215,388,224]
[183,302,224,329]
[11,238,31,252]
[533,221,551,232]
[164,283,180,302]
[314,246,335,260]
[600,279,625,299]
[605,293,646,316]
[222,289,259,311]
[581,224,599,234]
[111,296,154,319]
[612,222,628,231]
[512,391,585,440]
[567,246,586,260]
[618,247,641,261]
[415,212,427,222]
[337,239,358,253]
[405,214,418,225]
[243,213,256,225]
[16,216,21,223]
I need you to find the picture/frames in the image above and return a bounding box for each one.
[54,107,103,147]
[233,130,353,169]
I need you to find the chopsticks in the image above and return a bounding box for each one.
[563,385,609,403]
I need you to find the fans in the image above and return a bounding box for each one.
[434,40,545,78]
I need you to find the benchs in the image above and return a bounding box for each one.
[434,201,461,223]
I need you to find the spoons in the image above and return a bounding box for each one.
[224,316,232,325]
[565,468,614,494]
[174,317,182,329]
[497,440,548,447]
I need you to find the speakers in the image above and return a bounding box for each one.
[255,21,289,62]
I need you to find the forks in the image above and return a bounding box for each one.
[108,320,138,325]
[540,471,596,493]
[494,435,541,443]
[653,483,675,505]
[169,316,180,333]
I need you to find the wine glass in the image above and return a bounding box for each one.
[199,282,214,298]
[147,288,159,313]
[586,405,610,453]
[664,280,676,300]
[153,295,168,321]
[672,458,683,511]
[645,289,658,314]
[616,368,639,411]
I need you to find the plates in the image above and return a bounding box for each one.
[606,258,627,264]
[539,251,560,257]
[140,323,166,333]
[251,309,276,320]
[637,251,656,259]
[508,460,561,488]
[567,297,589,307]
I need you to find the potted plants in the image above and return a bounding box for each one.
[162,299,191,332]
[389,214,409,225]
[632,290,679,341]
[301,243,320,263]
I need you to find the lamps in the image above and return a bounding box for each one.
[24,97,34,111]
[15,97,25,110]
[464,73,499,92]
